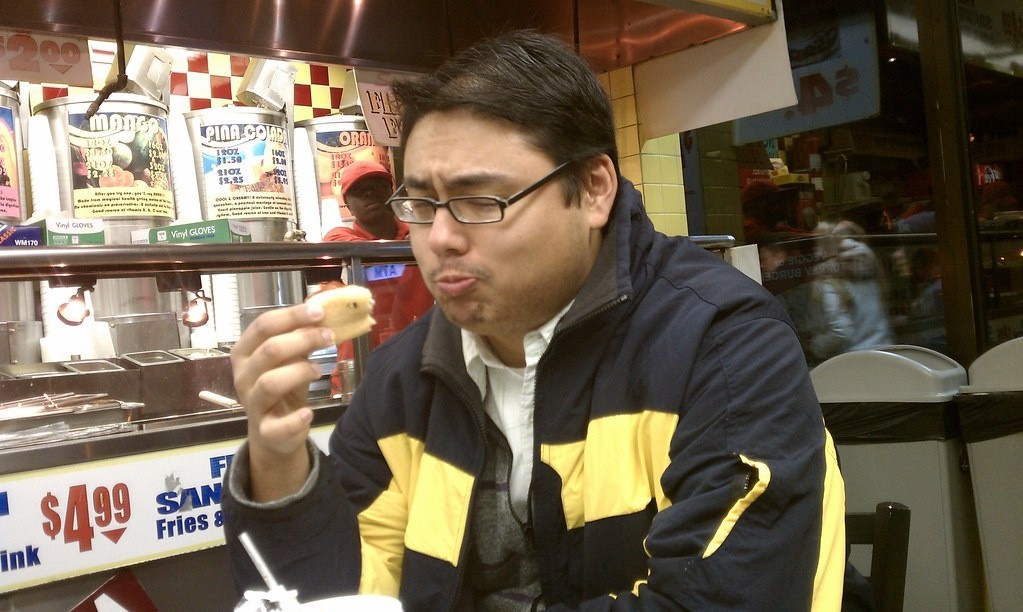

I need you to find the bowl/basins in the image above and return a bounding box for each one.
[15,345,234,381]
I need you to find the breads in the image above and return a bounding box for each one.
[306,285,377,343]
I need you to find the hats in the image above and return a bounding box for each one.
[341,160,396,204]
[740,181,790,205]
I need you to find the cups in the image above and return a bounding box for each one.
[302,594,404,612]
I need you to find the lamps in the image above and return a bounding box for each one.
[57,286,96,326]
[181,290,213,326]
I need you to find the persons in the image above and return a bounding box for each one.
[219,30,846,612]
[741,180,773,244]
[321,159,434,398]
[752,220,891,359]
[891,213,958,354]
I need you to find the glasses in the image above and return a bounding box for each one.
[384,160,574,224]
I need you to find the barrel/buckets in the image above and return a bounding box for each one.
[1,88,394,246]
